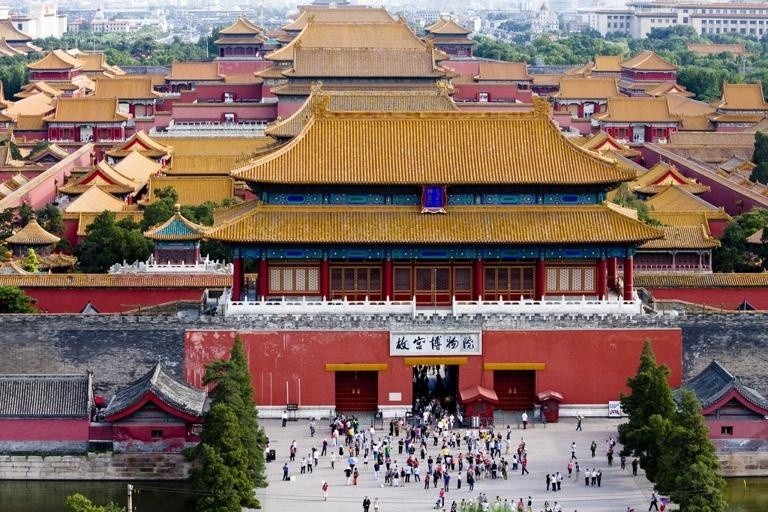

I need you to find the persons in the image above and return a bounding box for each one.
[264,364,672,511]
[736,199,744,215]
